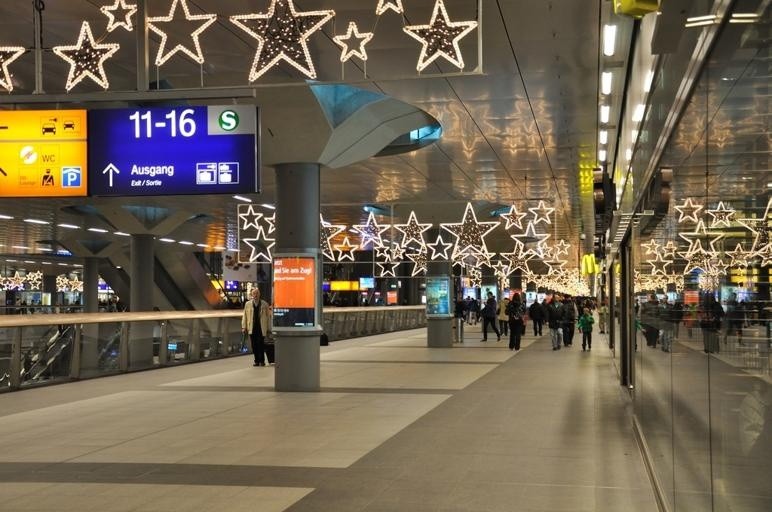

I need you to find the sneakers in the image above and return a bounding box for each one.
[480,333,508,343]
[509,345,521,350]
[553,340,592,351]
[534,330,543,337]
[252,362,274,368]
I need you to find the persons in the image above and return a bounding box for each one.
[16,297,22,315]
[21,300,28,315]
[241,287,273,367]
[454,289,606,352]
[635,287,772,354]
[56,293,122,334]
[29,299,36,314]
